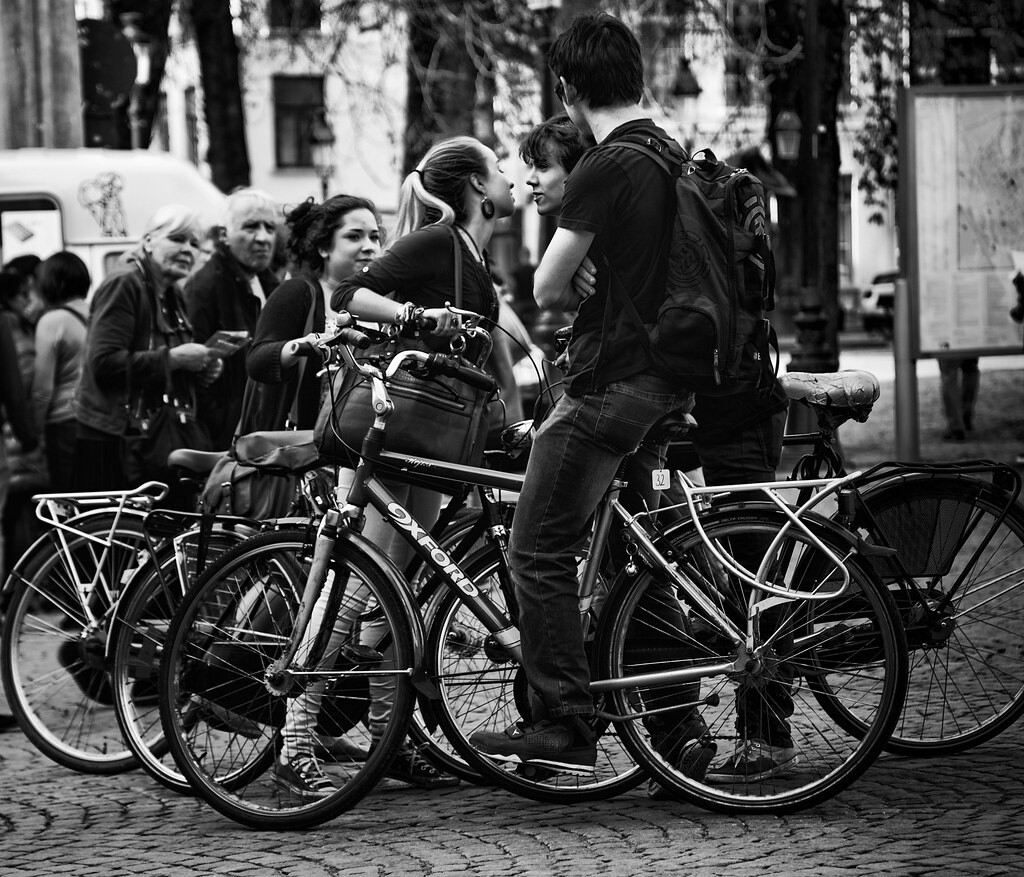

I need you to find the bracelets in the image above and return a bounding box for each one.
[393,302,415,323]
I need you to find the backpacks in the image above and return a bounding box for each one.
[591,134,781,397]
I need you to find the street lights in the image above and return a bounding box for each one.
[772,100,802,348]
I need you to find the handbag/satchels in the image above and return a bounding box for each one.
[119,346,217,515]
[313,340,491,495]
[194,454,300,528]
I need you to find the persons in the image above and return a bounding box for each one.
[517,109,800,783]
[469,12,719,802]
[268,136,516,802]
[936,353,981,443]
[234,196,385,764]
[0,184,281,739]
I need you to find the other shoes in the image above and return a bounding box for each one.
[189,693,261,739]
[270,753,338,803]
[131,676,192,707]
[366,739,460,789]
[942,424,976,441]
[314,736,371,762]
[0,713,19,732]
[57,637,118,706]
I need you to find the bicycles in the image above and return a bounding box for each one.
[0,291,1024,830]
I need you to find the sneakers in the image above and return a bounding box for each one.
[647,714,717,802]
[469,714,597,776]
[704,738,799,783]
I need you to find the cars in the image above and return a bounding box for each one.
[0,147,234,307]
